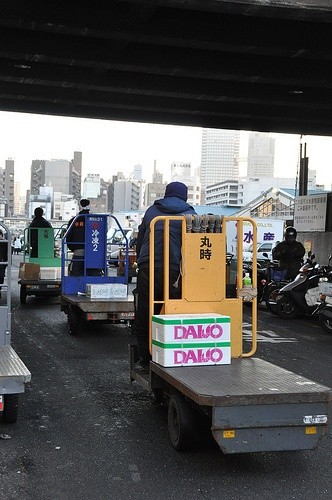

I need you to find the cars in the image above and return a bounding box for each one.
[107,227,140,266]
[243,239,280,262]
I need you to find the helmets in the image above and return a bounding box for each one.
[283,228,297,238]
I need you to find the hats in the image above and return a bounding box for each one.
[164,182,188,202]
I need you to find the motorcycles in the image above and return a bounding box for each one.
[260,250,332,334]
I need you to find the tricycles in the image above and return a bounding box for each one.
[128,214,331,456]
[60,214,135,329]
[18,227,68,305]
[0,221,32,425]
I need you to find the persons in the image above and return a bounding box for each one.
[0,229,8,299]
[272,226,306,281]
[135,181,199,364]
[28,207,55,258]
[11,235,22,255]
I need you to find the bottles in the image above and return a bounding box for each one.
[242,273,251,288]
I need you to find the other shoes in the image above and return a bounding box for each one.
[133,363,150,373]
